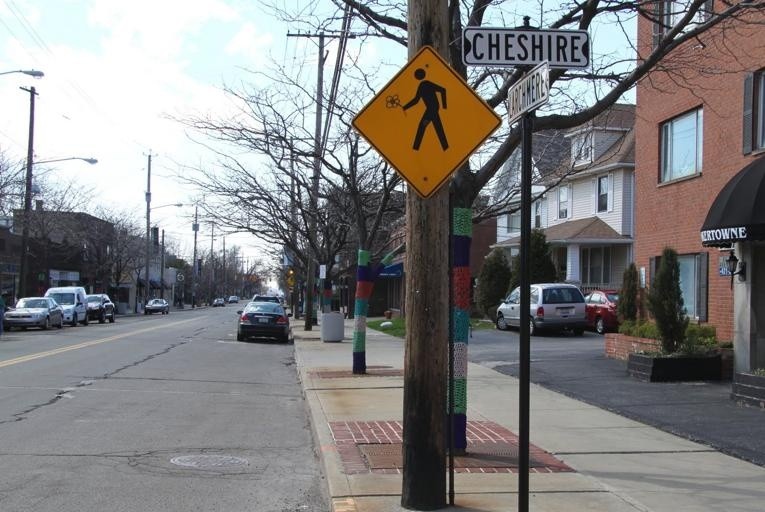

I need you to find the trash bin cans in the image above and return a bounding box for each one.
[118,303,127,314]
[321,311,345,342]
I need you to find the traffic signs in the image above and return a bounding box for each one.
[503,56,554,125]
[459,23,592,69]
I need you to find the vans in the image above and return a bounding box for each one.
[42,285,89,328]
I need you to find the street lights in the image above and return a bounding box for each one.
[144,203,185,311]
[0,68,44,77]
[17,156,98,298]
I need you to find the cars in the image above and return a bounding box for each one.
[226,297,241,303]
[211,297,225,307]
[1,295,64,332]
[580,286,625,333]
[228,295,238,304]
[142,298,169,316]
[494,281,589,338]
[233,294,294,344]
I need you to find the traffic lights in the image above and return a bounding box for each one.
[287,267,295,286]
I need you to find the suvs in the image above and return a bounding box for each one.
[83,293,115,325]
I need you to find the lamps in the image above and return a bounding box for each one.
[725,251,746,290]
